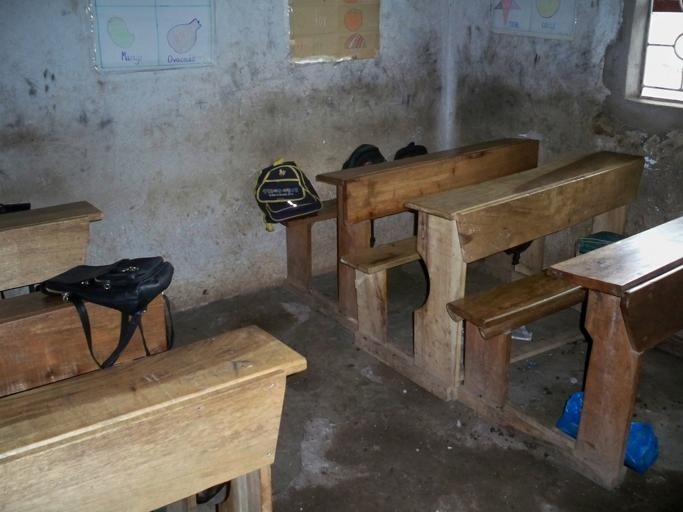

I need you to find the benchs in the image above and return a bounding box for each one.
[287,196,340,303]
[446,265,583,425]
[338,236,422,361]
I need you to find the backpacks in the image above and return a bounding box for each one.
[341,140,432,171]
[254,158,320,233]
[34,254,176,370]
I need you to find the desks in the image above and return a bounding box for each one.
[2,324,307,512]
[1,200,105,299]
[406,150,646,400]
[315,136,540,335]
[545,212,683,490]
[1,280,169,398]
